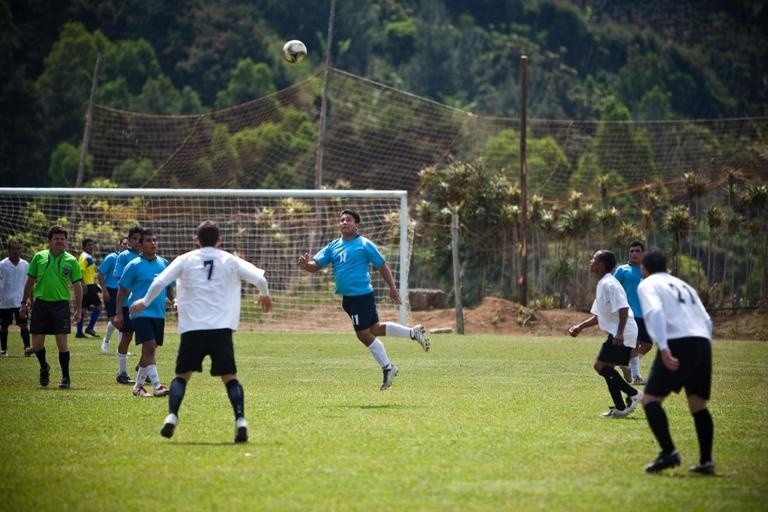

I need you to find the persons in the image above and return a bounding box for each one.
[112,227,177,397]
[95,235,132,357]
[111,224,155,386]
[613,240,652,385]
[74,238,105,341]
[18,226,84,389]
[297,206,431,391]
[127,218,276,444]
[637,246,715,476]
[0,236,34,357]
[566,250,645,420]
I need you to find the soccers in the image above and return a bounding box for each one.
[282,37,307,64]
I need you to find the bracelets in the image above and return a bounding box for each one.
[20,301,27,304]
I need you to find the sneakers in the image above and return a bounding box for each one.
[600,406,625,418]
[689,462,715,478]
[633,377,648,386]
[622,390,643,417]
[159,414,178,439]
[134,365,151,384]
[233,418,249,444]
[380,364,399,391]
[412,324,431,353]
[644,451,681,475]
[0,329,135,391]
[153,384,170,398]
[132,384,152,397]
